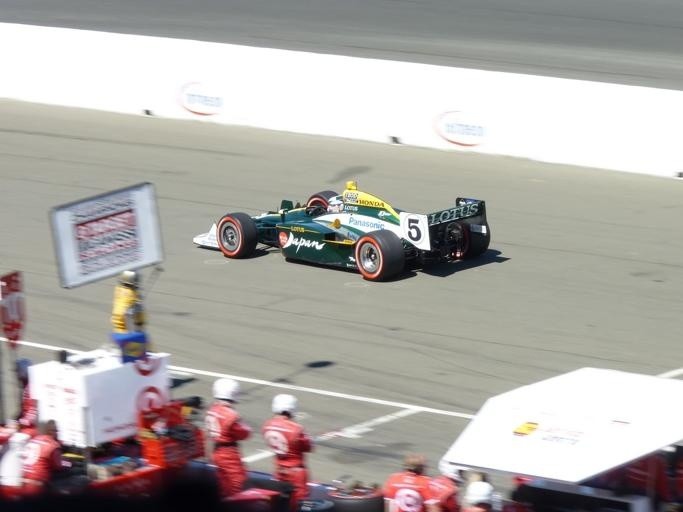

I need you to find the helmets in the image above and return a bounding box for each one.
[119,269,139,289]
[212,377,241,405]
[326,194,343,211]
[15,357,32,381]
[438,457,469,484]
[463,481,495,508]
[271,393,300,418]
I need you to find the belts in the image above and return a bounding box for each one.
[215,441,238,448]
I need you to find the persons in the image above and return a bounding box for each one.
[113,268,147,359]
[261,393,316,512]
[464,472,495,512]
[429,462,464,512]
[207,376,252,496]
[325,196,343,211]
[383,458,439,512]
[0,359,53,500]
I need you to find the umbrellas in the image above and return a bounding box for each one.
[443,367,683,485]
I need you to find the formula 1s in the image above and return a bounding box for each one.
[217,181,490,282]
